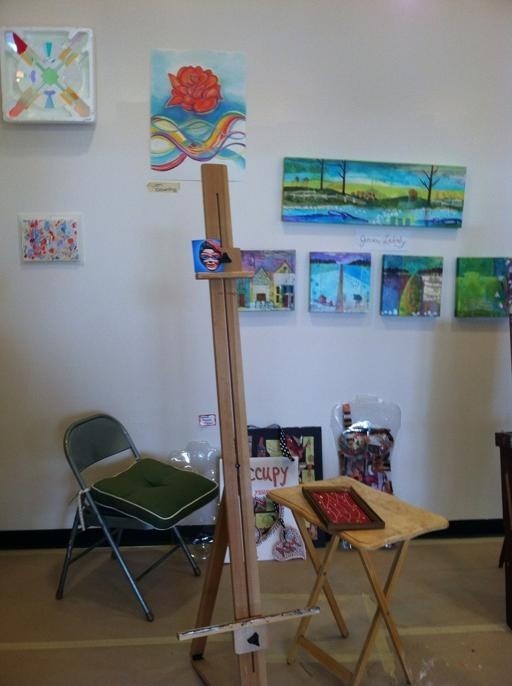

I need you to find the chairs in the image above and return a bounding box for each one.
[56,413,199,622]
[494,432,512,627]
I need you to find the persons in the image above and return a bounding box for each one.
[198,238,226,273]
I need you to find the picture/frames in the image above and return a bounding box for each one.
[248,427,325,547]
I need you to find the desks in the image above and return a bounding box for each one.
[268,476,449,686]
